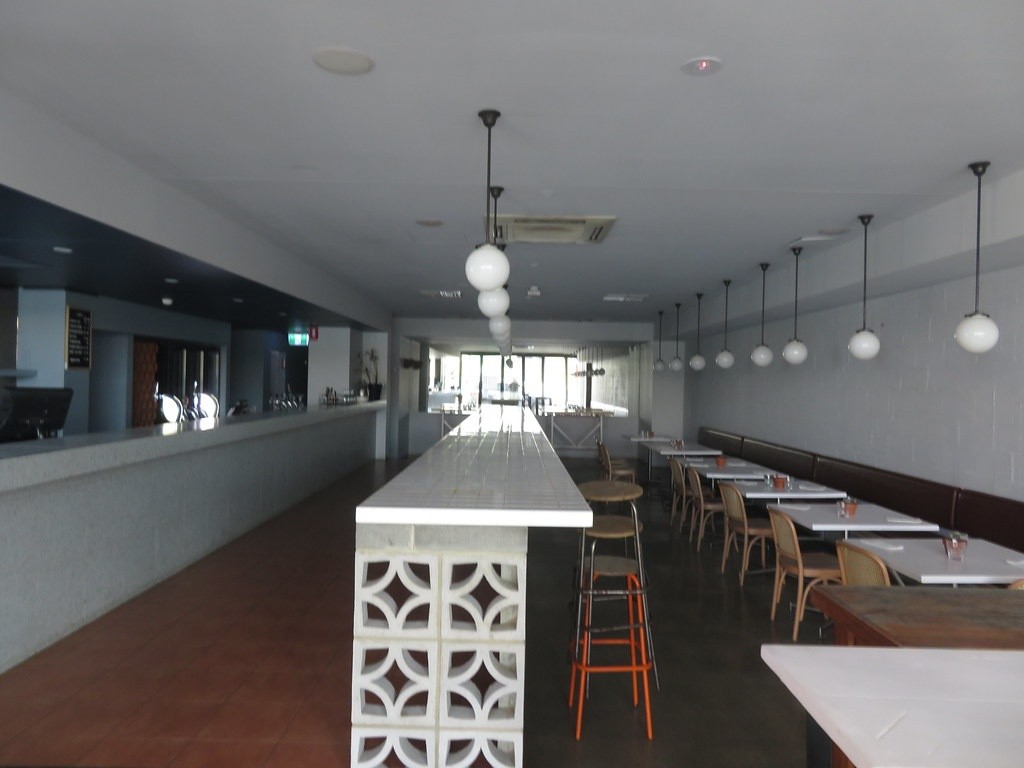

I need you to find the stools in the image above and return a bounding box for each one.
[568,480,661,741]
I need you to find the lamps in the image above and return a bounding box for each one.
[951,161,999,354]
[848,214,880,358]
[689,294,707,371]
[714,280,737,368]
[668,303,684,371]
[464,109,514,357]
[749,263,774,365]
[780,247,808,365]
[652,310,665,373]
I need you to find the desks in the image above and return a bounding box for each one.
[619,435,674,499]
[758,643,1024,768]
[811,582,1024,649]
[696,466,787,547]
[765,501,940,640]
[838,535,1024,587]
[349,404,594,768]
[720,478,847,580]
[674,454,747,534]
[642,440,723,513]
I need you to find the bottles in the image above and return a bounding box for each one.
[641,429,649,439]
[343,389,357,406]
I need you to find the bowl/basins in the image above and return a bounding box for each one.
[942,536,967,556]
[845,502,857,517]
[773,478,787,491]
[716,457,726,466]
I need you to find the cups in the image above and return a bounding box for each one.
[836,500,850,520]
[763,474,774,489]
[946,540,966,561]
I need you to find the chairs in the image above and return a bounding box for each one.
[667,458,713,527]
[684,465,721,553]
[766,508,841,642]
[595,442,635,504]
[716,479,775,586]
[834,539,891,587]
